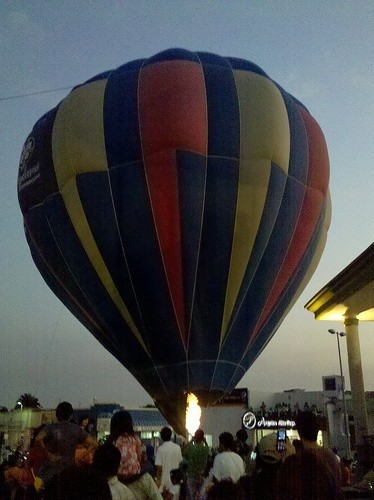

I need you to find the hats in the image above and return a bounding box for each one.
[254,432,298,465]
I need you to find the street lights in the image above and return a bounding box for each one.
[328,328,351,466]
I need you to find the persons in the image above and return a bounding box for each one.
[125,471,164,500]
[332,434,374,500]
[80,444,136,500]
[182,428,212,500]
[103,410,154,483]
[165,469,182,500]
[201,430,272,500]
[155,427,185,500]
[139,434,189,472]
[290,410,344,500]
[0,415,107,500]
[278,439,304,499]
[34,401,97,500]
[250,398,340,418]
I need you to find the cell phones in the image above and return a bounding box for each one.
[276,428,286,453]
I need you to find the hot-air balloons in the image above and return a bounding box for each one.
[17,48,333,441]
